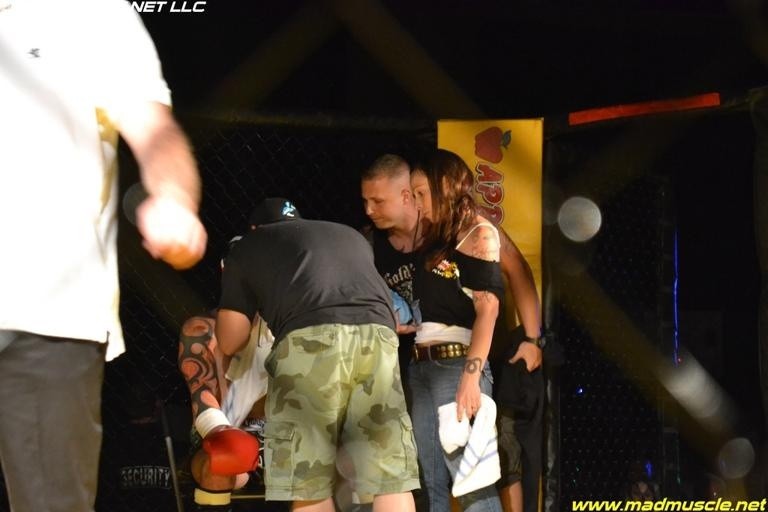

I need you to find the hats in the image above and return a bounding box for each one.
[248,197,301,225]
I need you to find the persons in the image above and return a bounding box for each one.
[0,1,210,512]
[178,147,547,512]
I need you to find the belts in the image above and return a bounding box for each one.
[411,342,470,364]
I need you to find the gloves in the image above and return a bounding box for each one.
[194,407,260,476]
